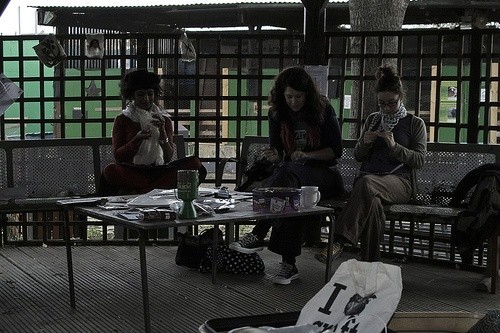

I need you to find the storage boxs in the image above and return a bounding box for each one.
[251,187,301,214]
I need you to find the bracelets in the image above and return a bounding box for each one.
[159,137,169,144]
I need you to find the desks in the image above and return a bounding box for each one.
[62,190,335,333]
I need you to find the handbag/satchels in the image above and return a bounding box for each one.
[198,245,265,277]
[296,259,403,333]
[175,228,224,268]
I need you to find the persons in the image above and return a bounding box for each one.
[103,70,202,195]
[227,66,342,285]
[87,39,101,55]
[313,65,427,264]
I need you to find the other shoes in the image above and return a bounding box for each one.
[457,253,473,269]
[477,277,492,293]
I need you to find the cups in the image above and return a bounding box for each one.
[300,186,321,209]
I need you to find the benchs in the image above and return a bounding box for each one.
[215,136,500,295]
[0,133,206,246]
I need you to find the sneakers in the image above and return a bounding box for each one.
[228,233,264,254]
[314,242,344,264]
[272,262,299,284]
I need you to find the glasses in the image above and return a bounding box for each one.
[377,96,401,107]
